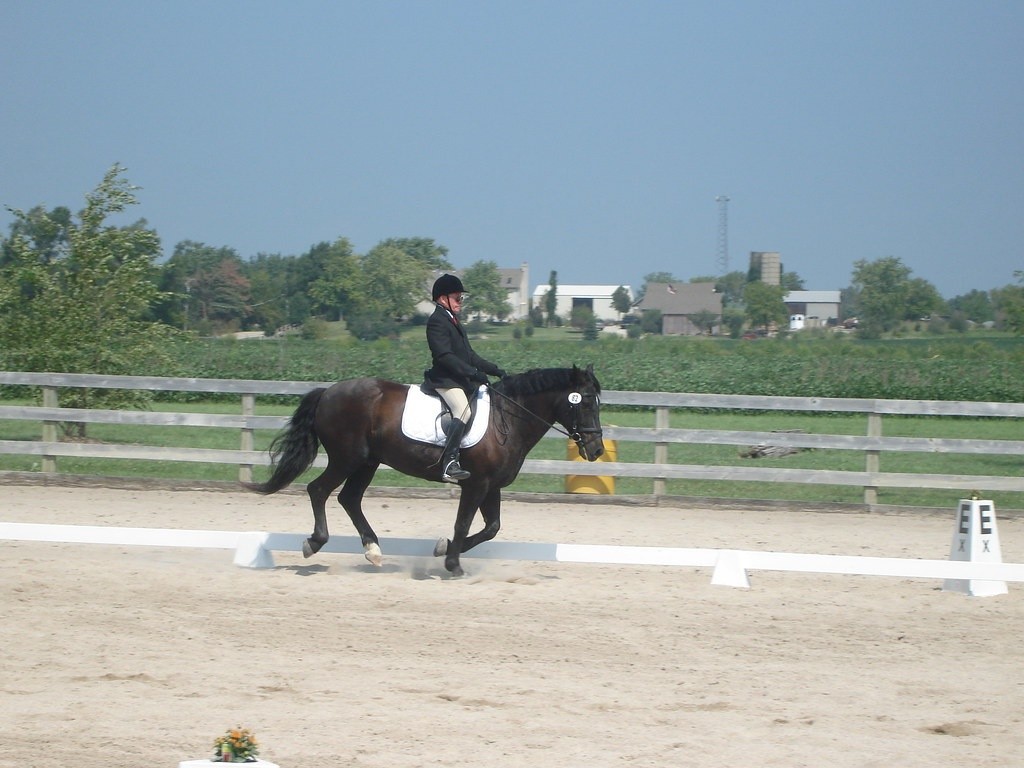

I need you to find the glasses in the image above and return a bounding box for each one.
[449,297,463,302]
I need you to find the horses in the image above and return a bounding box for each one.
[238,363,605,576]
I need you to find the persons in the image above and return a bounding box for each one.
[424,274,506,482]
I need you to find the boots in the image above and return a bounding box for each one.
[440,418,470,480]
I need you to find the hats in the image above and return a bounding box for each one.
[432,274,470,300]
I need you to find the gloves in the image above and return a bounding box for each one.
[472,370,489,384]
[495,369,506,378]
